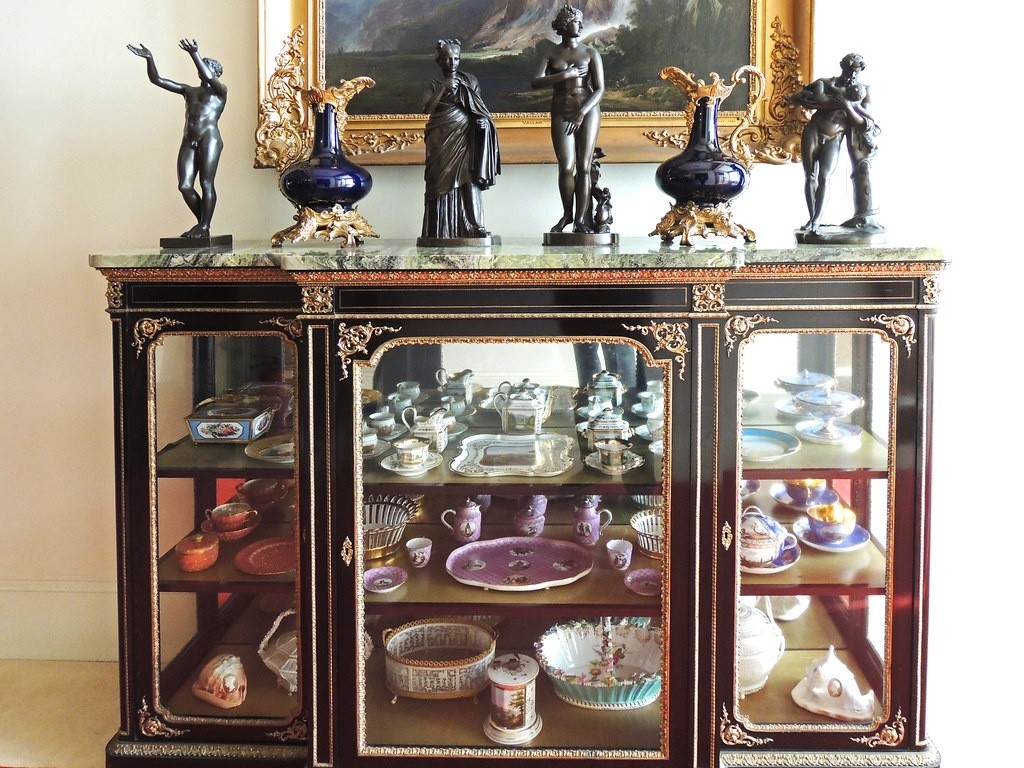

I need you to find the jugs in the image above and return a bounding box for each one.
[493,389,559,430]
[403,407,451,454]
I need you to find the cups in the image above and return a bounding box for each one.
[807,504,856,543]
[583,412,630,453]
[361,425,378,453]
[370,411,396,436]
[645,413,666,439]
[392,438,428,468]
[606,539,634,569]
[592,439,633,470]
[405,538,431,570]
[211,504,260,530]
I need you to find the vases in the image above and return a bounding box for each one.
[271,76,380,247]
[641,64,766,245]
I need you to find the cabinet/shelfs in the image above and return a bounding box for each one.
[86,241,950,768]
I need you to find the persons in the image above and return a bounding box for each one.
[789,53,874,235]
[126,39,227,238]
[422,38,501,238]
[531,5,611,231]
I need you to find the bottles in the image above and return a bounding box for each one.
[508,506,544,537]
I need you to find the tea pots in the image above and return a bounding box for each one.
[574,504,612,547]
[442,502,484,544]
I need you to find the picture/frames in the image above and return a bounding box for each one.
[254,0,816,167]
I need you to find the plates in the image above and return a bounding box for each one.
[387,380,669,419]
[244,411,801,478]
[200,500,871,596]
